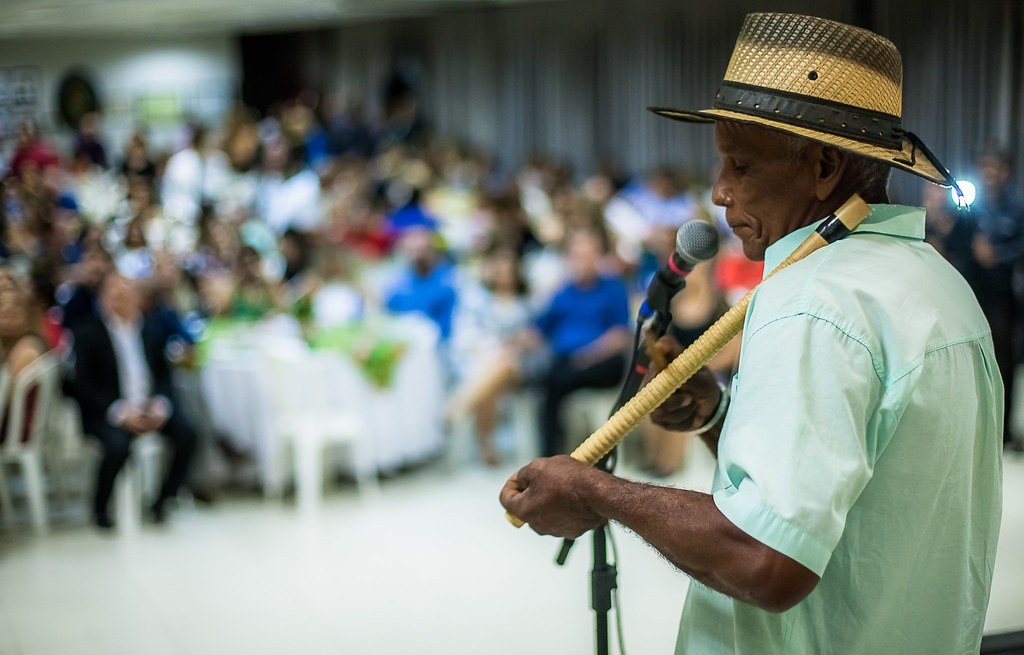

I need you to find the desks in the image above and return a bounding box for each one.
[198,331,440,520]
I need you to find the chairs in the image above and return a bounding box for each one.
[0,346,66,540]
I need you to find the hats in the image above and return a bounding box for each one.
[646,13,971,212]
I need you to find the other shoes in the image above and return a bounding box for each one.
[151,502,161,520]
[92,506,113,528]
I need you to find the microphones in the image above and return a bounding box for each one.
[637,220,719,323]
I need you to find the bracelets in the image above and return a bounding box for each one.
[689,381,728,434]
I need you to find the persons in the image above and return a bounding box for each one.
[501,11,1004,654]
[0,66,1024,533]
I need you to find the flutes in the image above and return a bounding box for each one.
[503,188,878,532]
